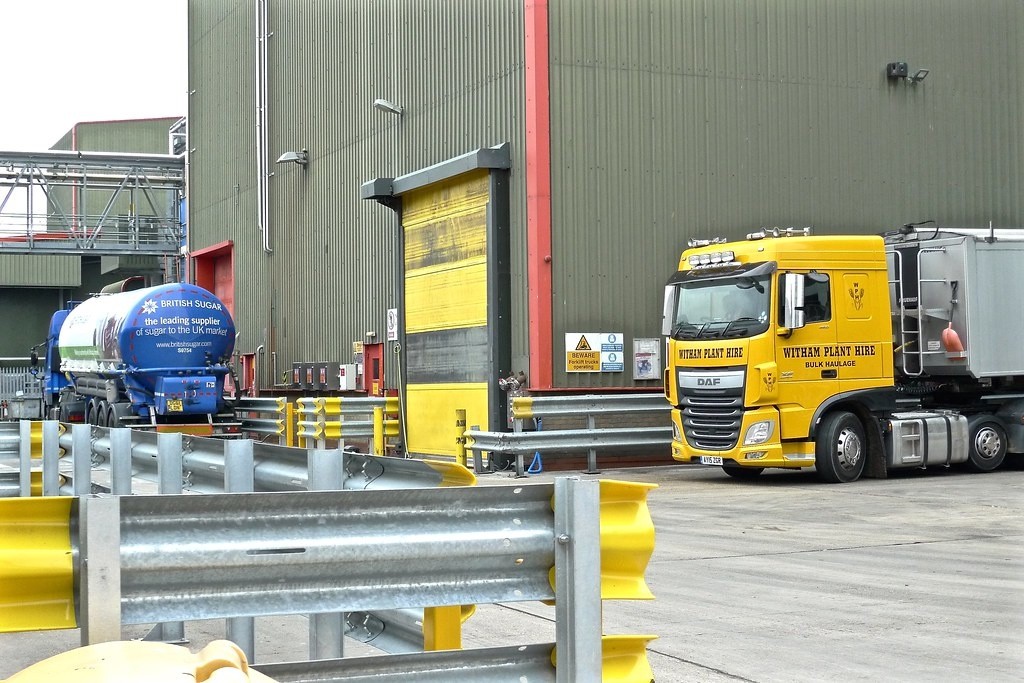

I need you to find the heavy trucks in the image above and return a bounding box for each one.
[660,218,1024,485]
[28,281,244,438]
